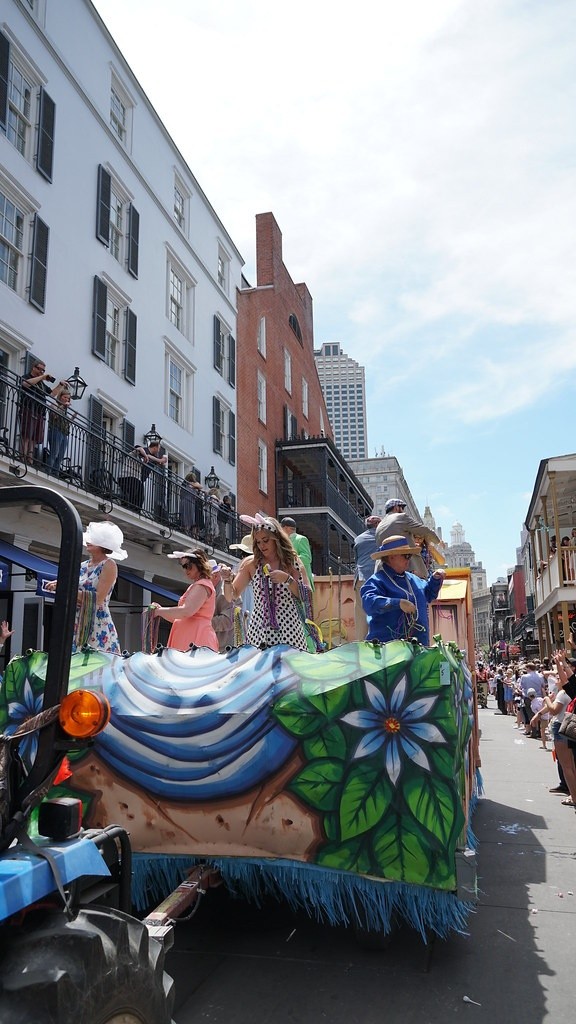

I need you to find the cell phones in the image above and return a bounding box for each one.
[46,375,55,383]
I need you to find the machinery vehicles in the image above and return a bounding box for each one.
[0,482,180,1024]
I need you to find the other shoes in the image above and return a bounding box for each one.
[561,799,575,805]
[482,704,553,753]
[549,785,568,793]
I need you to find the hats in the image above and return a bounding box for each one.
[82,521,128,560]
[366,517,381,525]
[385,498,406,512]
[370,536,421,560]
[229,535,254,553]
[206,559,223,575]
[565,656,576,667]
[527,688,536,697]
[280,517,296,528]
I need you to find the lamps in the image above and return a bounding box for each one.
[143,424,163,447]
[26,504,42,513]
[152,543,162,555]
[65,366,88,399]
[205,466,219,488]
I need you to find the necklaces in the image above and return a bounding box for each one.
[379,568,425,640]
[197,574,204,580]
[292,583,323,653]
[143,608,159,653]
[76,590,96,646]
[234,610,243,644]
[257,561,279,628]
[434,581,453,620]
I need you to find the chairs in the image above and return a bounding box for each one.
[1,423,186,533]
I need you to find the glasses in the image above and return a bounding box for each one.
[183,560,193,569]
[402,553,412,560]
[35,366,45,372]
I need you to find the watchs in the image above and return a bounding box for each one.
[283,576,294,585]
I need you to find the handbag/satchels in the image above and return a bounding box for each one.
[559,707,576,741]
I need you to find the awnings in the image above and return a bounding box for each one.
[0,541,58,598]
[109,571,181,614]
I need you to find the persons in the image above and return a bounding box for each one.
[360,535,446,646]
[280,517,315,592]
[229,535,253,641]
[220,514,312,651]
[119,442,167,512]
[538,528,576,587]
[45,522,128,654]
[476,649,576,805]
[142,549,219,651]
[19,361,79,475]
[354,516,382,641]
[180,473,231,550]
[208,559,242,651]
[0,621,15,652]
[374,499,445,580]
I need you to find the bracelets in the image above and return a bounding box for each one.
[284,575,290,583]
[225,580,232,584]
[538,712,542,716]
[60,382,63,386]
